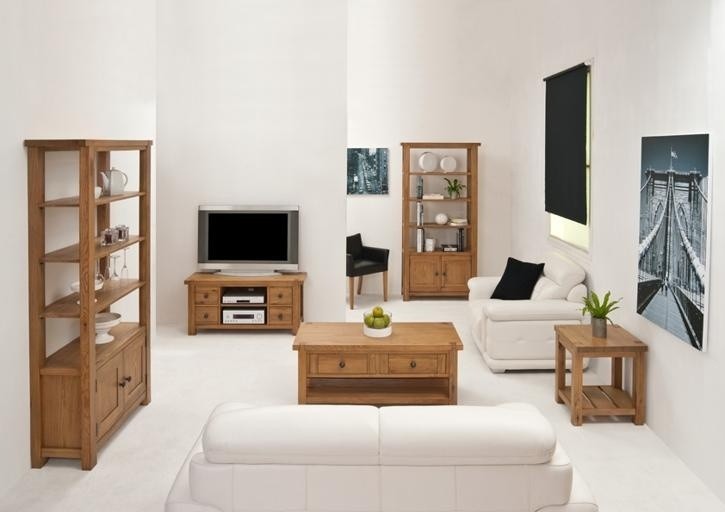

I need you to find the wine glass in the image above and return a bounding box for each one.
[94,247,130,291]
[93,311,121,344]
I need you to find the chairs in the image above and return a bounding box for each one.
[346,233,390,310]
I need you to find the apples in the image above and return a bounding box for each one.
[373,307,383,317]
[365,316,374,327]
[384,315,389,327]
[373,318,385,329]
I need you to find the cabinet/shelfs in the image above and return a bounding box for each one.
[401,139,481,301]
[184,272,307,335]
[23,138,155,470]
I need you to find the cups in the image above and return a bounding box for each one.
[99,223,130,246]
[93,187,102,200]
[425,239,436,252]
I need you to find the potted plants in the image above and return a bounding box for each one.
[443,177,467,201]
[580,290,625,337]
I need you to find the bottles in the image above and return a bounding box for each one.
[416,228,424,252]
[415,202,423,226]
[416,176,423,199]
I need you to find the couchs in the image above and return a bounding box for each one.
[466,252,592,373]
[164,402,599,511]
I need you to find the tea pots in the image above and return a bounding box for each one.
[100,166,128,197]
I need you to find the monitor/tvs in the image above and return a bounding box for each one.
[197,205,299,276]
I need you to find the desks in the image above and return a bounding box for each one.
[554,324,649,425]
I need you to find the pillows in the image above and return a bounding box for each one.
[490,258,545,301]
